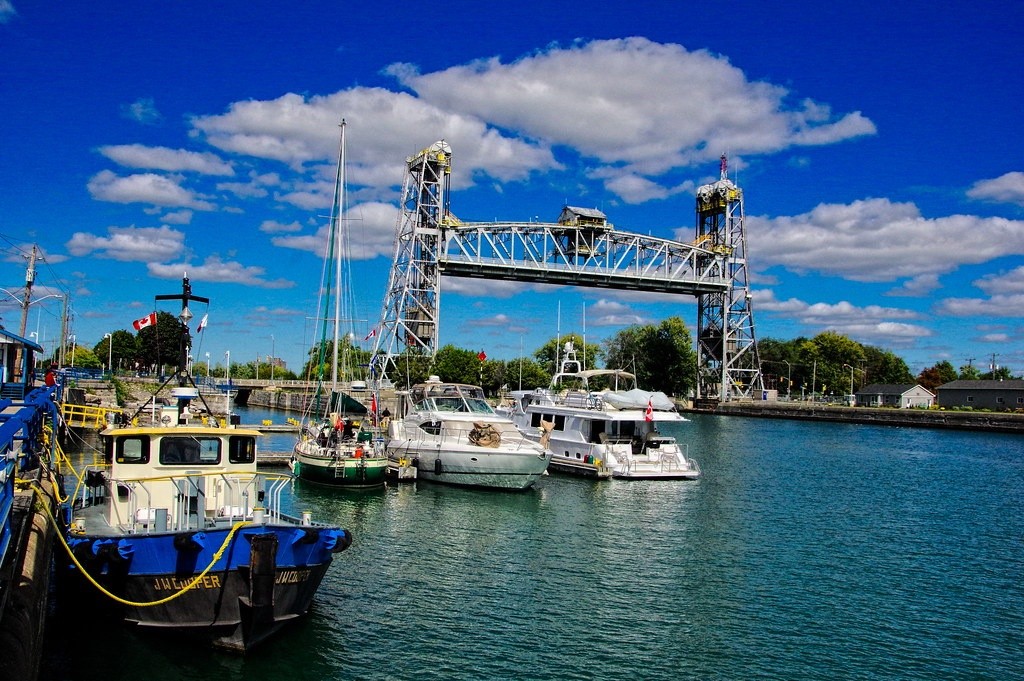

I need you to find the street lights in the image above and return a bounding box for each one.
[0,288,62,384]
[69,334,76,368]
[29,332,38,369]
[844,364,854,407]
[206,352,210,385]
[267,354,273,380]
[782,360,790,402]
[225,351,230,415]
[271,334,275,380]
[104,333,113,370]
[185,346,189,370]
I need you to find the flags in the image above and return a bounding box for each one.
[365,330,376,340]
[132,313,156,330]
[645,400,653,422]
[196,314,208,333]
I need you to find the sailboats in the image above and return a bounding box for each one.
[288,118,391,489]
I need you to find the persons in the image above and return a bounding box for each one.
[344,421,355,438]
[44,364,55,387]
[60,366,66,377]
[32,365,37,386]
[382,407,391,417]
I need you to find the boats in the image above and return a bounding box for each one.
[349,381,367,392]
[191,351,239,415]
[68,426,354,650]
[380,381,551,490]
[412,383,487,400]
[496,336,702,481]
